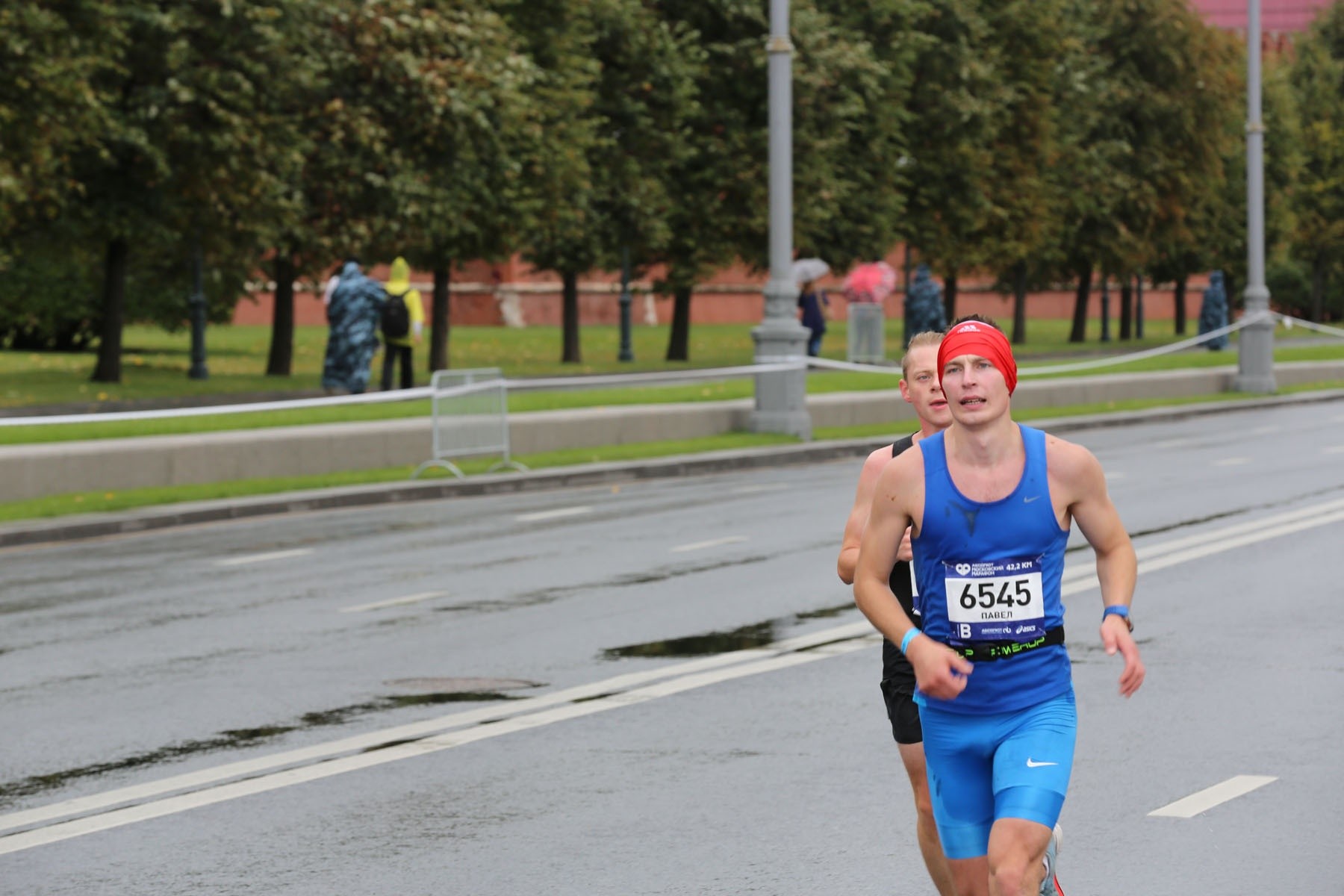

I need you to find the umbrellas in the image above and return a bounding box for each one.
[841,261,898,306]
[793,258,831,284]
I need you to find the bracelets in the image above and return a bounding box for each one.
[1101,604,1134,633]
[900,626,922,656]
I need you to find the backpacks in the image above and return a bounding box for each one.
[381,287,412,338]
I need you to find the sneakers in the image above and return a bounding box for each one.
[1039,822,1064,896]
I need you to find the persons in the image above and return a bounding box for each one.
[797,282,831,357]
[903,263,947,350]
[1197,271,1230,351]
[846,297,885,364]
[836,330,1063,895]
[852,313,1147,896]
[322,255,426,396]
